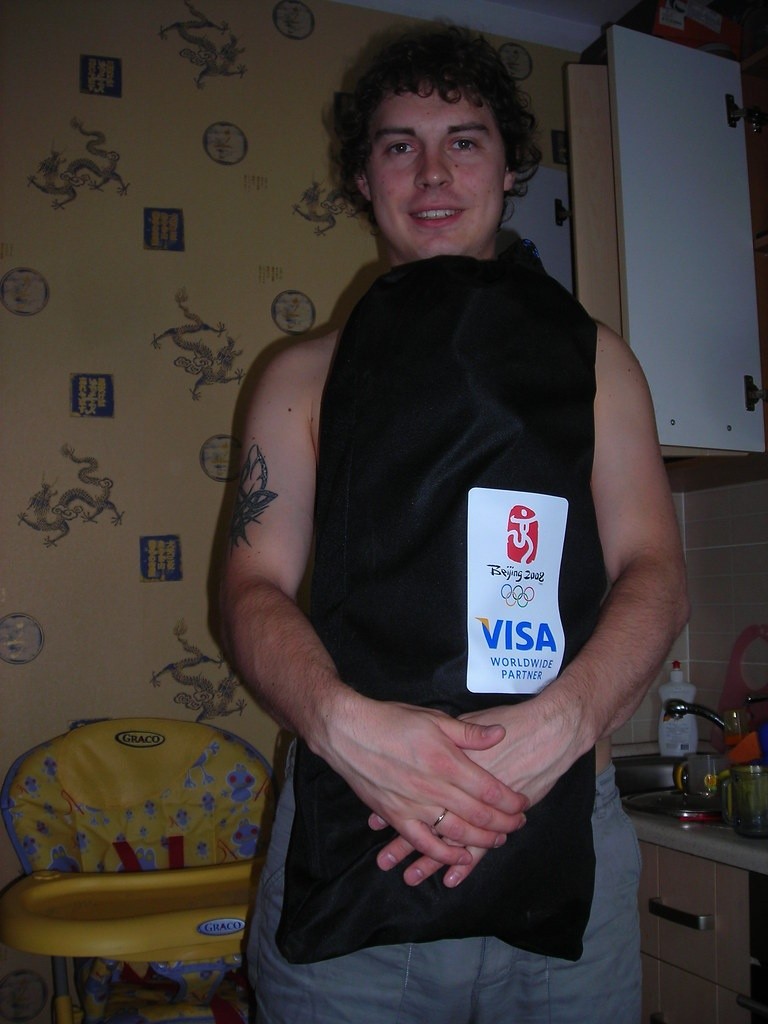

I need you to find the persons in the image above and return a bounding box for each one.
[216,19,689,1024]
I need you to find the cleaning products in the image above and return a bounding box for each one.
[655,658,700,759]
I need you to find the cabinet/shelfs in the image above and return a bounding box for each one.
[635,840,768,1024]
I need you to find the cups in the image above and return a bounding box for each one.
[720,764,768,839]
[676,752,727,811]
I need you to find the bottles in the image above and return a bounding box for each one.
[656,659,699,760]
[723,709,757,765]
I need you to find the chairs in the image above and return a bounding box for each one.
[0,717,279,1024]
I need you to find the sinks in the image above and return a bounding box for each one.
[611,760,698,800]
[630,790,687,809]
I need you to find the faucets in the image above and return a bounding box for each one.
[662,697,727,732]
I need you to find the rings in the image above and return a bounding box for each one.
[434,808,448,826]
